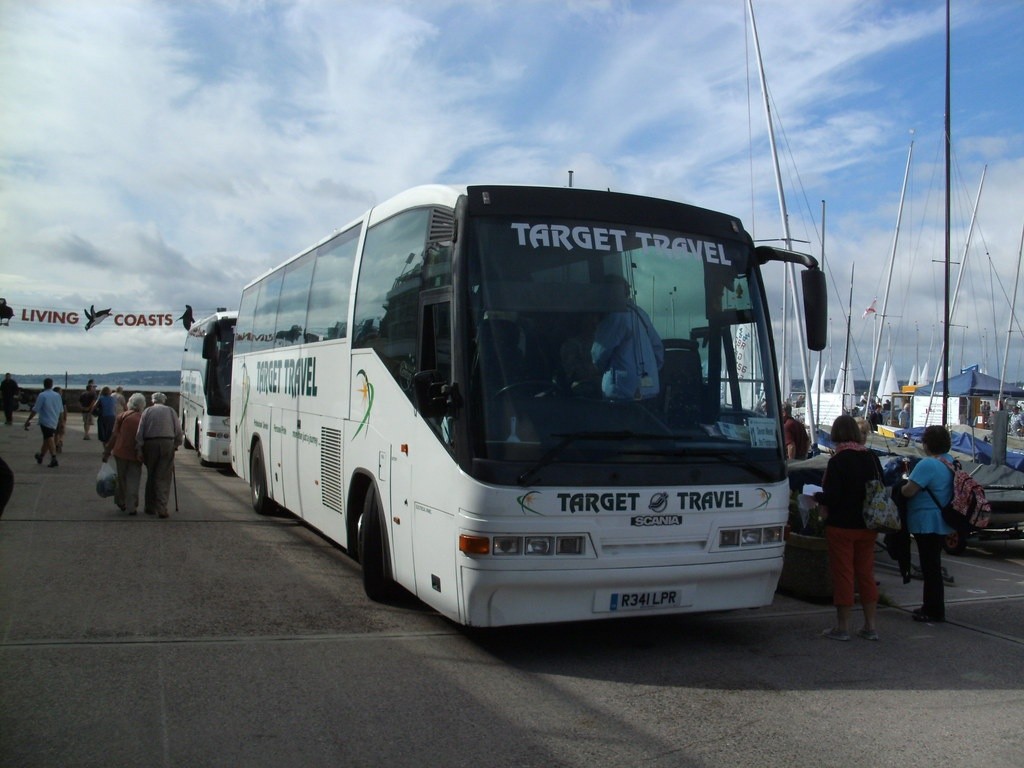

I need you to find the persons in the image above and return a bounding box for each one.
[134,392,183,518]
[795,395,802,409]
[591,274,664,401]
[25,378,64,467]
[93,387,117,448]
[786,395,793,403]
[860,392,867,402]
[811,414,883,640]
[901,425,957,623]
[869,405,883,431]
[111,387,127,413]
[980,400,990,415]
[898,403,910,428]
[1010,405,1024,433]
[102,393,146,515]
[782,402,810,514]
[53,387,68,447]
[80,379,97,440]
[0,373,19,424]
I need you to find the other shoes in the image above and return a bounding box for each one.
[912,606,944,622]
[129,510,136,515]
[47,459,58,467]
[160,512,169,518]
[119,503,125,510]
[856,626,878,642]
[84,436,90,440]
[821,627,852,641]
[56,441,63,454]
[35,451,43,464]
[145,508,156,516]
[5,420,13,426]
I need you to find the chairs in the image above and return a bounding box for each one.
[484,318,525,401]
[658,338,705,424]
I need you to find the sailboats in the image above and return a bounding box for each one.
[718,1,1024,551]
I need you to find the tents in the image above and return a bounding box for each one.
[915,369,1024,425]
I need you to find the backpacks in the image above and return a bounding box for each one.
[926,455,991,533]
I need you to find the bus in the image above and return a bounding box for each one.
[227,184,830,631]
[178,310,239,466]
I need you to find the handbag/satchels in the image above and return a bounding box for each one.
[96,461,119,498]
[863,453,903,535]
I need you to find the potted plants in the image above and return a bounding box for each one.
[783,488,858,604]
